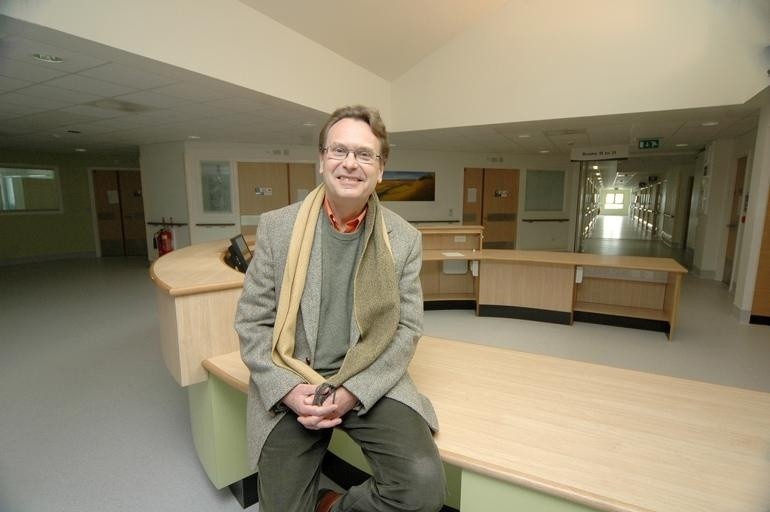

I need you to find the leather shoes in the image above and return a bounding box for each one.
[315,488,345,512]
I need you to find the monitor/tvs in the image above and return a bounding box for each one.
[228,234,252,272]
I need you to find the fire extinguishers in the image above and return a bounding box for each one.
[152,228,172,257]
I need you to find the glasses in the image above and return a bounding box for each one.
[320,145,382,164]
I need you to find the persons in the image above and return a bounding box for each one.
[233,106,447,512]
